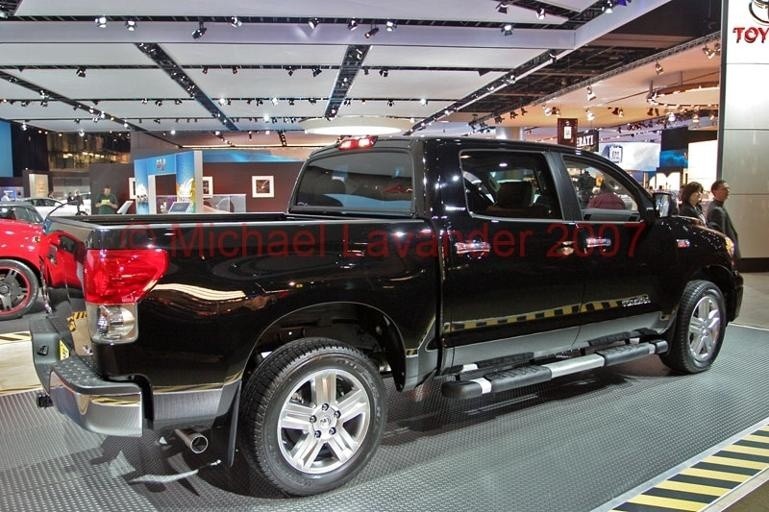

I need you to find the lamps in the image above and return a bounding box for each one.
[191,18,207,39]
[365,20,379,38]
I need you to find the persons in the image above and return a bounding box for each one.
[586,180,742,261]
[94,185,119,214]
[0,190,84,206]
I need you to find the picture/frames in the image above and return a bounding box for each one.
[202,176,213,198]
[129,178,136,199]
[252,175,274,198]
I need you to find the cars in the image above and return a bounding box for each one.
[0,202,45,323]
[16,198,89,220]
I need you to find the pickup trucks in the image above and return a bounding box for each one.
[28,134,744,498]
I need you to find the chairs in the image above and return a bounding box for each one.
[485,179,544,218]
